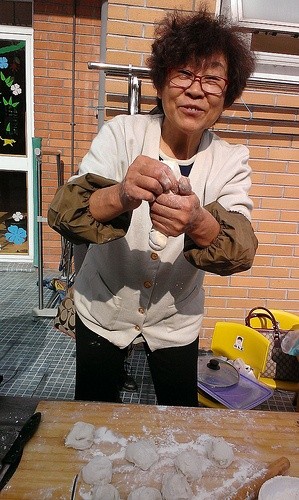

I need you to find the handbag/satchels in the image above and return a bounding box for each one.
[245,307,299,381]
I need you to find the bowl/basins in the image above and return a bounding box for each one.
[258,476,299,500]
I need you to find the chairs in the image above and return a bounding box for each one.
[198,322,270,409]
[250,308,299,406]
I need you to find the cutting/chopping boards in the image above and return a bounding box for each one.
[0,400,299,500]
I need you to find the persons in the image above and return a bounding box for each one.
[47,1,258,409]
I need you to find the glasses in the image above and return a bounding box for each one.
[167,68,228,94]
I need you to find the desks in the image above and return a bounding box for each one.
[0,400,299,500]
